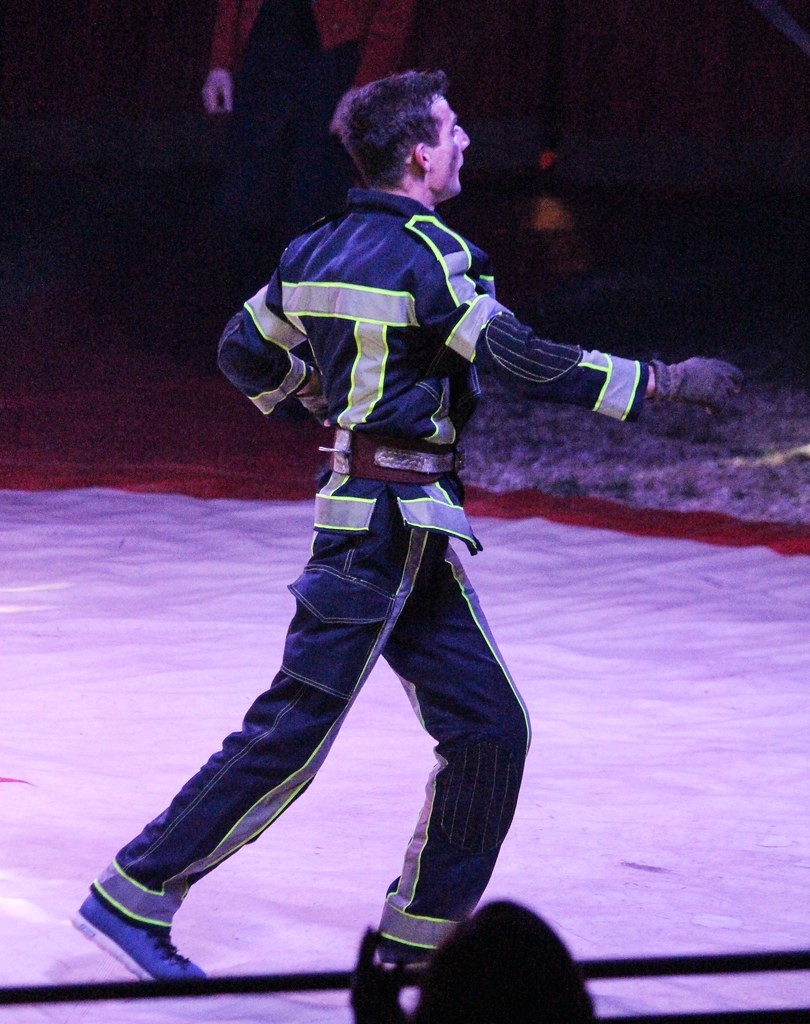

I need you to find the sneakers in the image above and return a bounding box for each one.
[377,937,434,970]
[71,893,206,981]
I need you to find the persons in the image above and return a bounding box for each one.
[85,67,741,977]
[351,898,596,1023]
[204,1,416,229]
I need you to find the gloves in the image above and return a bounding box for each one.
[295,361,330,427]
[649,357,744,415]
